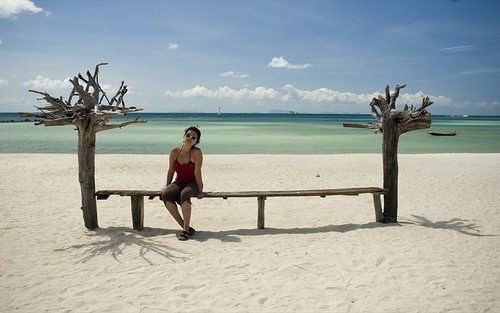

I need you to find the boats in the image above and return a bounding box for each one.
[427,129,458,136]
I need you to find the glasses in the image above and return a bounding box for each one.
[186,133,197,141]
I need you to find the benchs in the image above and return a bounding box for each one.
[92,186,390,230]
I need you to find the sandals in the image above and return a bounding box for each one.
[189,227,195,235]
[179,231,189,240]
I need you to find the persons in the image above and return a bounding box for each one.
[159,125,204,242]
